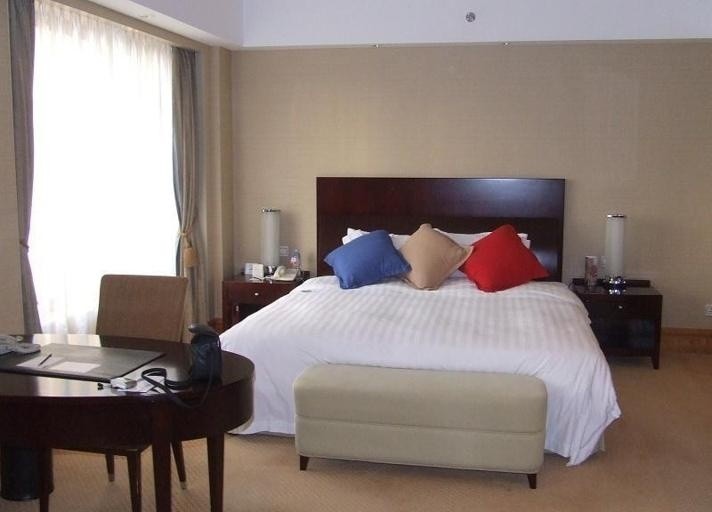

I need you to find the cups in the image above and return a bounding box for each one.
[585,256,599,290]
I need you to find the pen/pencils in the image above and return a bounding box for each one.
[39,354,52,366]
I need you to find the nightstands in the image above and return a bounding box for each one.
[223,267,310,325]
[573,279,663,370]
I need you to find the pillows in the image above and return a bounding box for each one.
[321,223,550,292]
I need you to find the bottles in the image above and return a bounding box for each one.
[291,248,302,278]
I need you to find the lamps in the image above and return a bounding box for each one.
[603,214,627,295]
[261,209,285,280]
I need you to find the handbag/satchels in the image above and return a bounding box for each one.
[185,322,226,383]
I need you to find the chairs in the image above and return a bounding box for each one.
[36,274,200,511]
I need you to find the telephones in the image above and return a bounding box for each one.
[272,266,299,281]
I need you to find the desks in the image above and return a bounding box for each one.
[0,336,256,511]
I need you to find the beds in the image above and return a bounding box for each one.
[218,176,623,468]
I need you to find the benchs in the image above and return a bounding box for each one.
[293,364,549,489]
